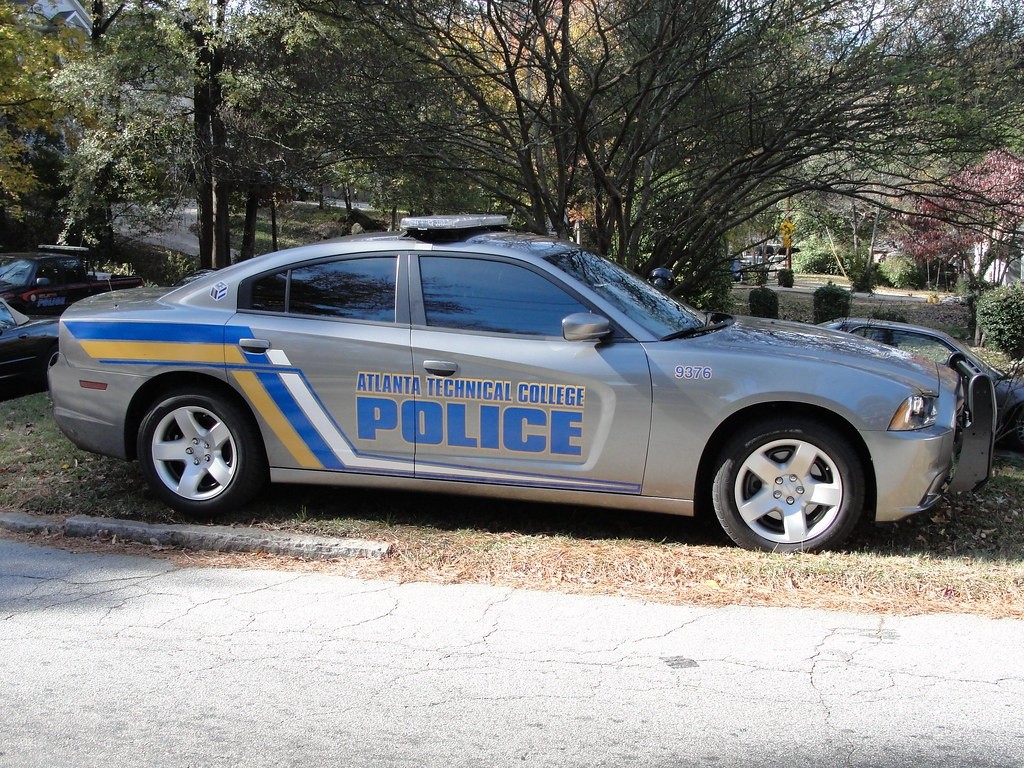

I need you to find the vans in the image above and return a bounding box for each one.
[754,244,800,263]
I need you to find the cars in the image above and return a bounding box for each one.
[45,208,998,555]
[0,297,60,389]
[817,316,1024,455]
[726,254,749,283]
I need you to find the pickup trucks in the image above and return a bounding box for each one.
[0,244,145,316]
